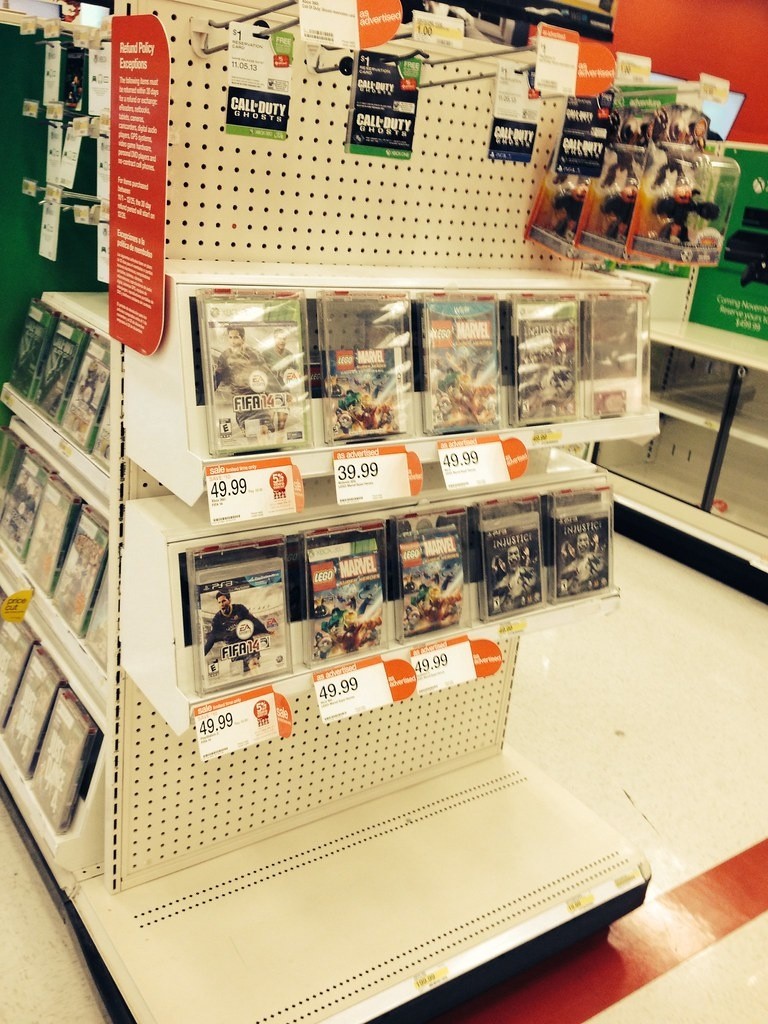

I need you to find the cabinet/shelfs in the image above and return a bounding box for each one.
[0,0,768,1024]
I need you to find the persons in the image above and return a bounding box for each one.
[12,319,107,437]
[210,323,300,443]
[203,587,275,674]
[484,530,542,614]
[558,519,608,592]
[3,470,39,556]
